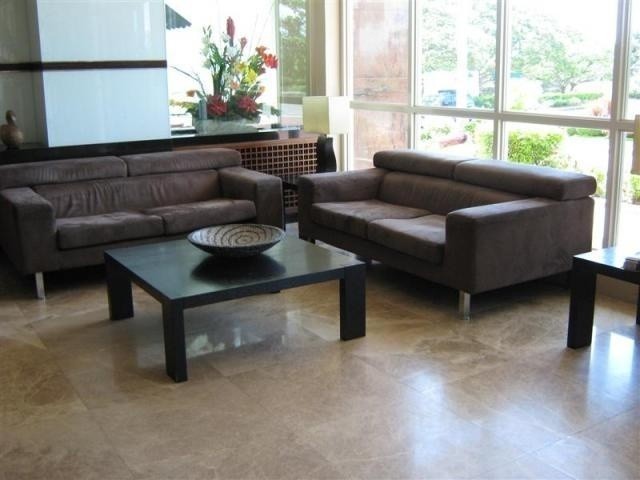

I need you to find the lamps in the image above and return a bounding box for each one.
[302,95,348,172]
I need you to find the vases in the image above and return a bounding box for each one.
[192,117,258,137]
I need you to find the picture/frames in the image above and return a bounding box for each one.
[276,0,311,105]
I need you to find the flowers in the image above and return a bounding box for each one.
[170,16,281,122]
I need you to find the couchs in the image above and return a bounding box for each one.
[1,149,284,300]
[297,150,597,321]
